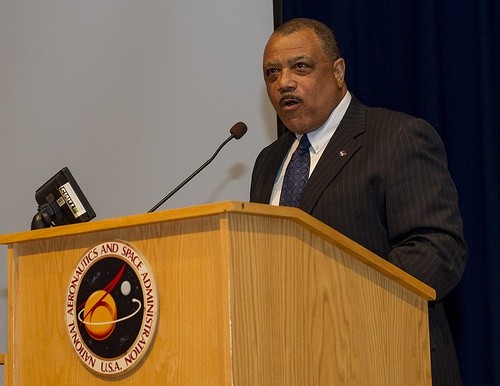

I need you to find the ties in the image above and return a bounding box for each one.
[279,133,312,207]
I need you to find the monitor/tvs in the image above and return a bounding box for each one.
[35,167,97,228]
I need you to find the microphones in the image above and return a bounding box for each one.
[146,121,249,212]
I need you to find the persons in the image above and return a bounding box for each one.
[248,18,469,386]
